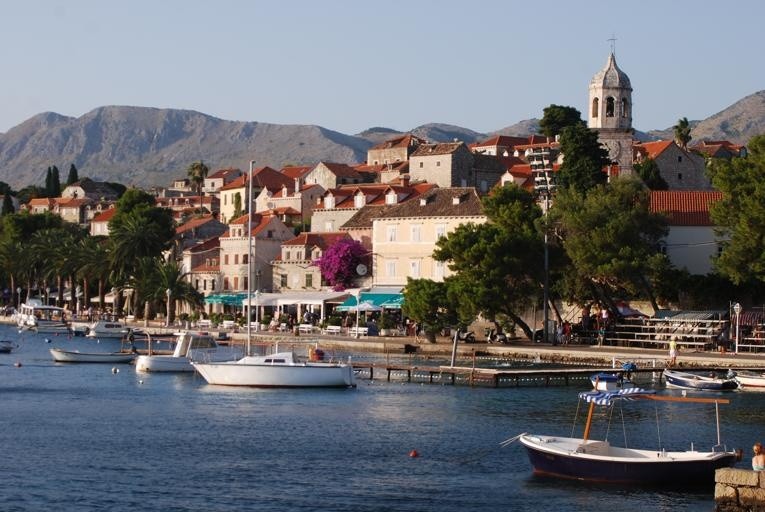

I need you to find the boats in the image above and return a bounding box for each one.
[519,368,765,489]
[0,306,243,387]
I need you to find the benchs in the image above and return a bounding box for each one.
[199,320,368,337]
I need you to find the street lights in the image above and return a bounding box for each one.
[354,290,362,341]
[46,287,50,306]
[111,287,116,322]
[252,288,261,332]
[76,286,80,317]
[540,186,550,344]
[734,303,742,353]
[166,289,172,326]
[17,287,21,307]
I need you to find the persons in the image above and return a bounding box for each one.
[669,334,680,365]
[600,305,609,330]
[751,443,765,471]
[581,304,592,328]
[412,321,421,344]
[556,320,563,343]
[2,302,113,325]
[563,320,571,337]
[597,327,605,347]
[287,309,353,337]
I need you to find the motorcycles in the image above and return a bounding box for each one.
[452,328,475,343]
[484,328,507,345]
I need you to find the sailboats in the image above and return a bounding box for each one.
[190,160,358,388]
